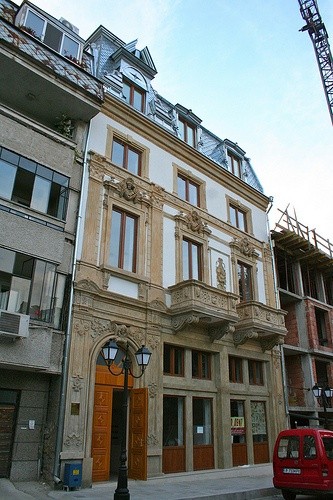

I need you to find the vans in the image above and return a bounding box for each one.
[272,428,333,499]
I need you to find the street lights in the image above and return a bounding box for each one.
[311,382,333,429]
[99,339,153,500]
